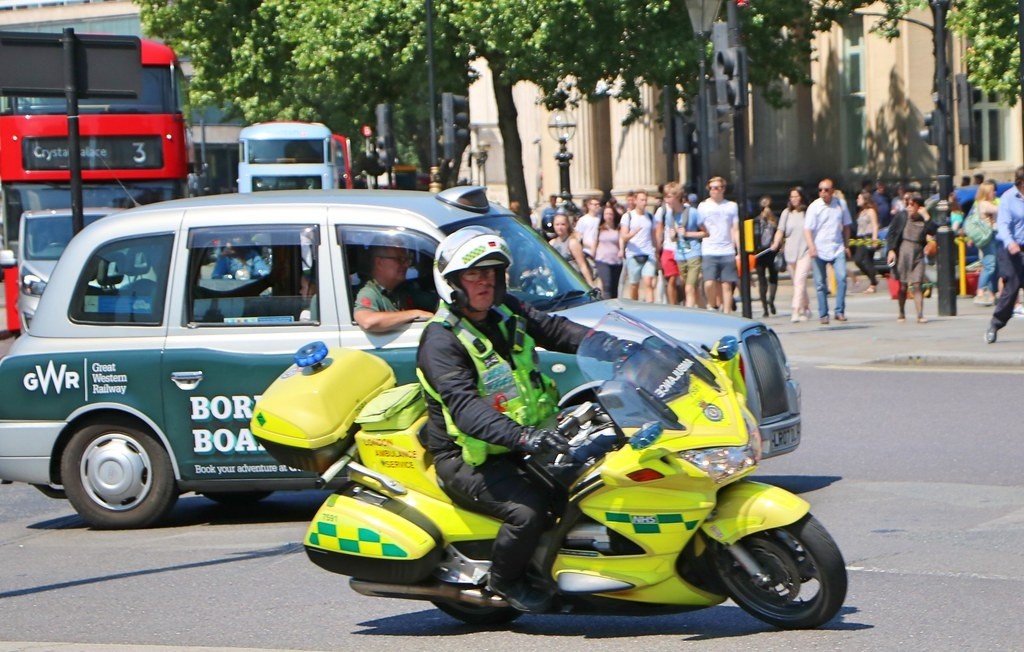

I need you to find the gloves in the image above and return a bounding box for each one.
[514,429,568,458]
[604,339,641,361]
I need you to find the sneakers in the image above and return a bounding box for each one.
[488,571,551,613]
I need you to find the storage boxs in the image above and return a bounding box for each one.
[303,480,444,584]
[250,347,397,474]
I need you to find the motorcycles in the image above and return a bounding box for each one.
[249,309,847,631]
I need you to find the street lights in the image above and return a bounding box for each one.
[547,108,576,200]
[685,0,723,200]
[818,5,957,316]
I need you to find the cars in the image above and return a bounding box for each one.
[872,183,1015,276]
[0,207,159,335]
[0,184,802,530]
[354,156,430,190]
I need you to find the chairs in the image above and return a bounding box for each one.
[347,244,365,302]
[124,245,159,321]
[82,246,126,320]
[311,294,317,321]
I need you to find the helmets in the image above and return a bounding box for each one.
[432,226,511,306]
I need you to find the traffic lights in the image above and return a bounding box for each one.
[441,92,470,160]
[361,124,373,137]
[918,110,944,146]
[708,106,730,152]
[716,46,748,107]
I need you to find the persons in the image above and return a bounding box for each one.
[359,135,392,188]
[985,165,1024,343]
[300,226,317,294]
[416,224,644,611]
[186,163,201,199]
[771,187,814,323]
[299,268,318,323]
[352,235,436,333]
[753,195,788,317]
[211,234,271,285]
[803,179,853,323]
[508,176,743,322]
[827,173,1001,307]
[885,193,938,323]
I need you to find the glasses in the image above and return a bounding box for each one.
[709,186,725,192]
[818,188,831,193]
[452,268,496,282]
[376,254,414,267]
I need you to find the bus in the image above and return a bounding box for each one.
[0,31,188,257]
[237,122,332,194]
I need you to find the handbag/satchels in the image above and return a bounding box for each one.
[964,205,994,250]
[886,266,901,298]
[774,251,786,272]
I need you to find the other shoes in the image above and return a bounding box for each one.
[766,299,776,315]
[986,325,997,343]
[916,316,927,323]
[821,314,830,325]
[834,312,847,323]
[973,295,997,307]
[898,314,905,323]
[801,304,810,320]
[866,284,877,293]
[791,309,799,323]
